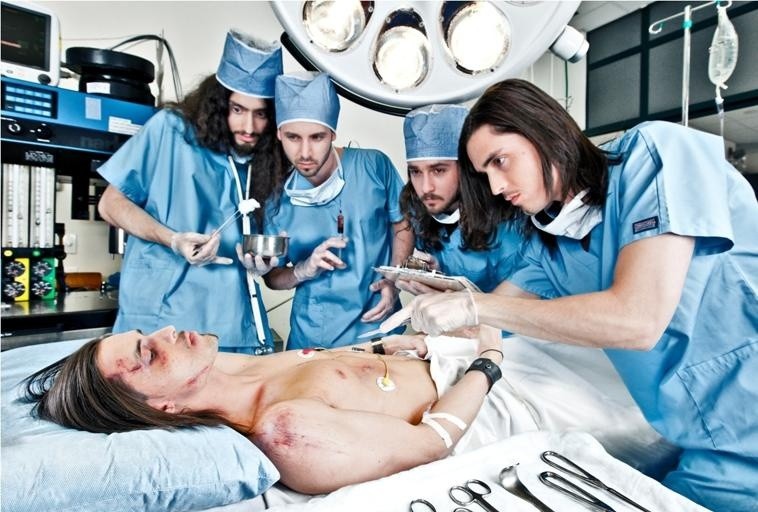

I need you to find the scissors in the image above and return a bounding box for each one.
[449,479,502,512]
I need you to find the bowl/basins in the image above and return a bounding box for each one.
[242,234,290,260]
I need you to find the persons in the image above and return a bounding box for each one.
[95,27,282,353]
[398,105,535,293]
[259,71,417,351]
[394,76,758,512]
[21,326,684,495]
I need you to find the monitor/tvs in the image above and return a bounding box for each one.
[0,1,62,88]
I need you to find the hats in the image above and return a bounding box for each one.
[215,27,286,98]
[274,71,341,133]
[402,104,469,161]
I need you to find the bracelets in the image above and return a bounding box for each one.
[477,348,504,361]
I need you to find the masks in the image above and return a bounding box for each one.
[529,185,605,241]
[282,147,346,209]
[431,206,462,226]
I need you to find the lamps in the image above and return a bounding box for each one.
[373,26,434,91]
[445,2,514,76]
[301,0,366,52]
